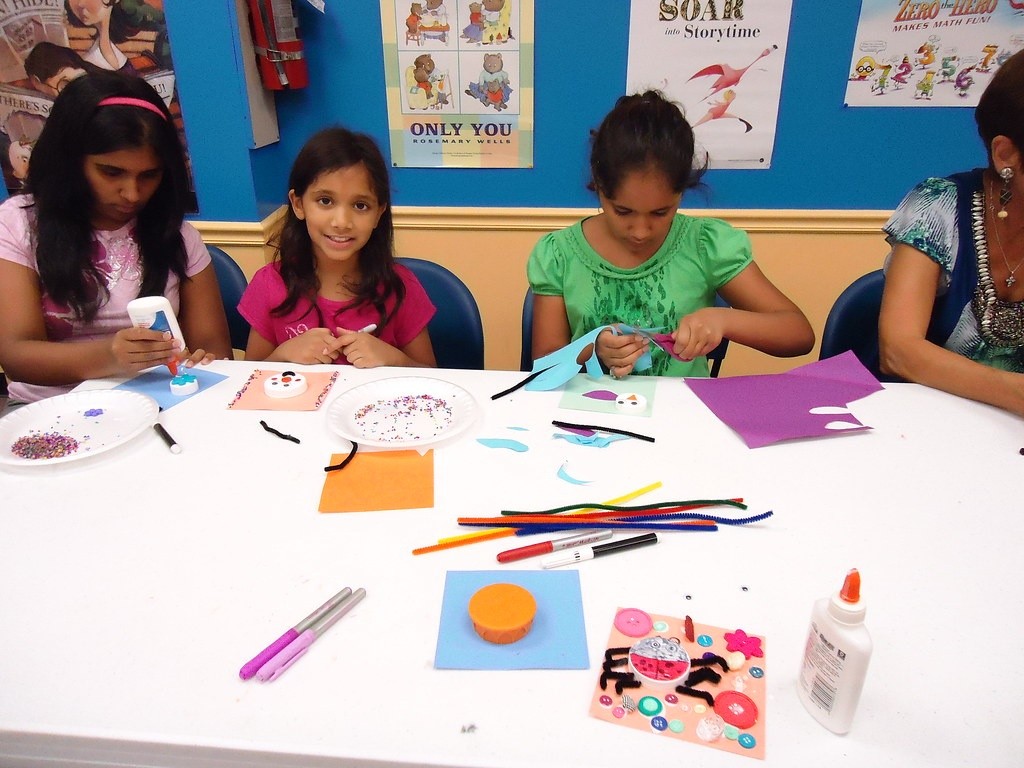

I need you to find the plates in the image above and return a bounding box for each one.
[0,389,159,464]
[326,376,480,447]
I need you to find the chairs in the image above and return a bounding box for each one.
[205,249,889,386]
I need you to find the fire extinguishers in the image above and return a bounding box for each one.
[249,0,309,97]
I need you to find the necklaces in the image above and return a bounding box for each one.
[989,172,1024,288]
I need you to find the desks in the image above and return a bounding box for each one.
[0,355,1024,767]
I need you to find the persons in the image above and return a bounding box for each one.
[526,89,816,378]
[0,71,235,418]
[236,126,440,369]
[879,49,1024,416]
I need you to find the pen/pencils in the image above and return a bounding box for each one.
[152,420,182,454]
[258,588,366,682]
[239,586,352,680]
[359,324,377,334]
[540,532,658,570]
[496,528,614,564]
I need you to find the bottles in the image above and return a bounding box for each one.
[797,570,872,737]
[127,296,185,376]
